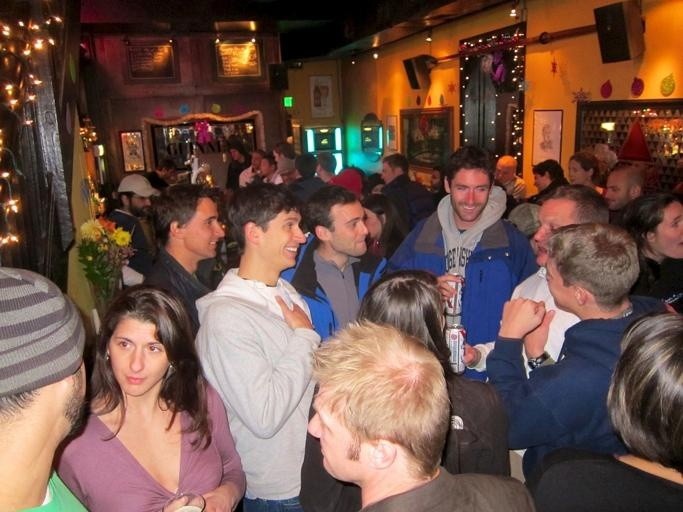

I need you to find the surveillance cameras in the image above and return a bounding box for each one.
[285,57,307,71]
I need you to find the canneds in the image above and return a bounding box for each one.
[444,323,467,376]
[444,272,466,317]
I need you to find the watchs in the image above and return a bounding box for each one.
[528,351,550,369]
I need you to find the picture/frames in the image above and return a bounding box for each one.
[119,129,146,174]
[123,40,181,84]
[401,107,454,172]
[531,109,563,167]
[212,39,265,82]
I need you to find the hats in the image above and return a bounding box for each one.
[1,267,86,397]
[118,175,161,197]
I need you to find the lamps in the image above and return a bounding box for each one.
[426,31,431,42]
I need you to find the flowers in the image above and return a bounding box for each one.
[76,216,136,324]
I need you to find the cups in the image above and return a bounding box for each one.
[162,492,206,512]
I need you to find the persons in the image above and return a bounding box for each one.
[438,185,608,487]
[108,139,682,346]
[0,267,86,512]
[55,284,246,512]
[535,315,683,512]
[486,222,670,489]
[299,268,508,511]
[279,185,387,512]
[194,183,323,512]
[539,123,553,150]
[306,318,535,511]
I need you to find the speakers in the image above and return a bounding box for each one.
[592,0,646,65]
[267,63,289,91]
[401,54,432,92]
